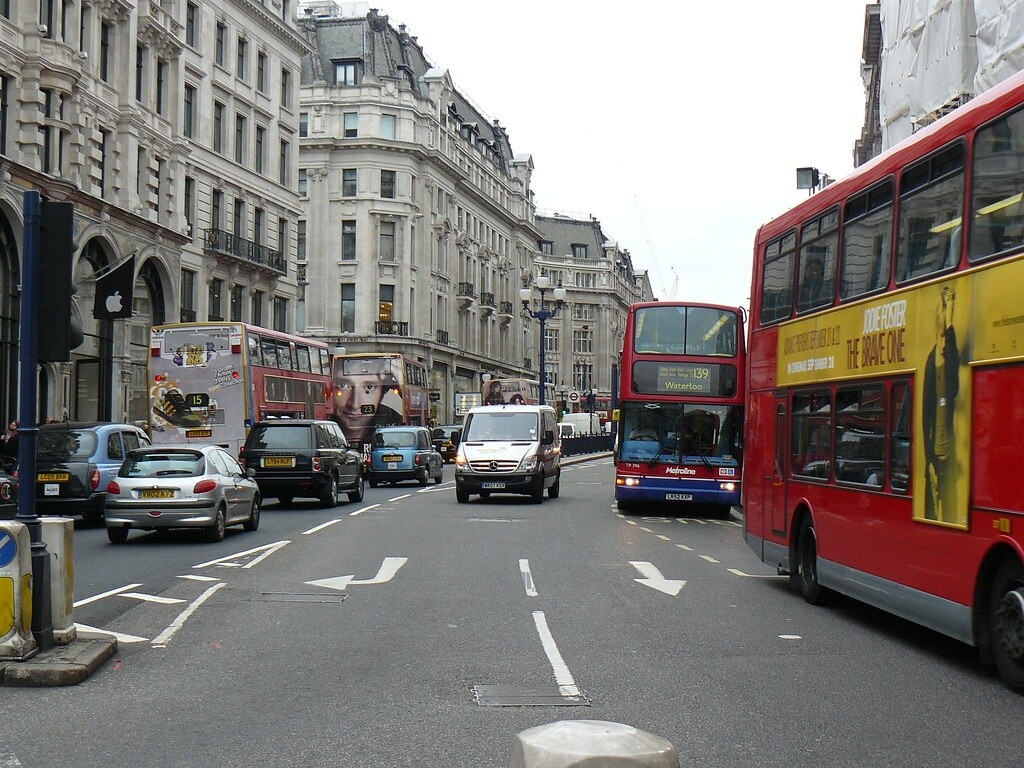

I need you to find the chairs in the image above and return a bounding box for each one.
[150,456,170,469]
[836,443,870,471]
[811,427,840,448]
[636,429,659,440]
[950,216,994,266]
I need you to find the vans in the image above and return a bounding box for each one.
[558,414,606,447]
[0,420,153,525]
[455,401,562,505]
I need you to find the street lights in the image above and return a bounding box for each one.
[592,383,598,413]
[575,356,586,404]
[519,267,567,406]
[560,380,567,416]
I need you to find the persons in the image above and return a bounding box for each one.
[485,381,525,405]
[923,285,962,522]
[334,357,403,443]
[0,418,75,478]
[628,412,659,441]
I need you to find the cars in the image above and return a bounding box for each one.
[101,442,262,543]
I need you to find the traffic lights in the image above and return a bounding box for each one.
[36,200,85,363]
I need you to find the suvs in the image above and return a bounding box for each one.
[430,425,464,462]
[365,425,445,488]
[237,418,365,507]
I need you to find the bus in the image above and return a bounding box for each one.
[332,351,431,448]
[481,378,557,422]
[143,320,336,464]
[572,394,619,426]
[605,300,748,521]
[736,69,1024,694]
